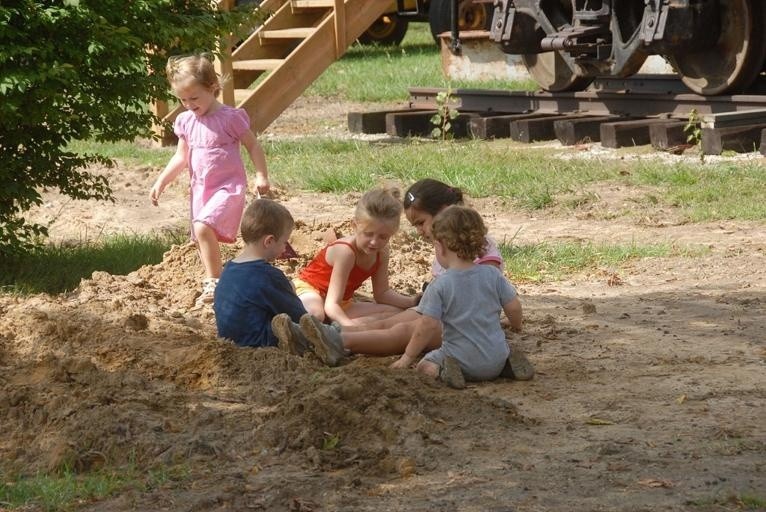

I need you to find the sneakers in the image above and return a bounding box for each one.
[272,313,311,355]
[440,356,464,387]
[510,348,534,381]
[203,278,218,303]
[299,314,351,365]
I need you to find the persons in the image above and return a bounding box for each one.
[149,51,269,305]
[289,191,422,328]
[271,178,502,368]
[390,205,535,388]
[213,199,308,348]
